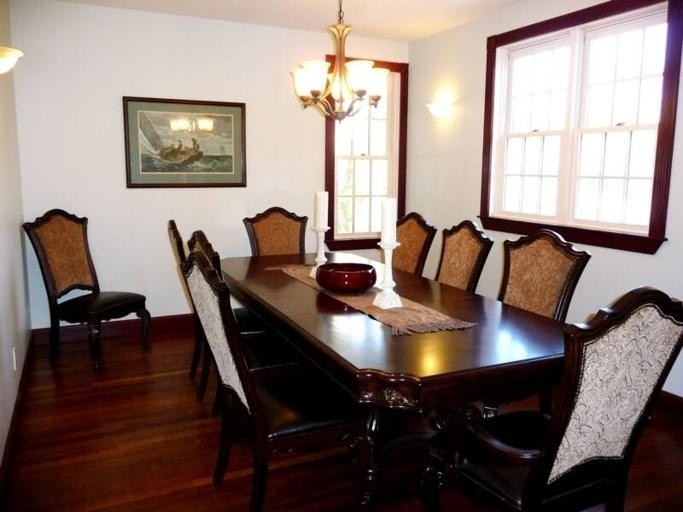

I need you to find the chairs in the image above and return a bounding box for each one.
[21,208,152,372]
[168,206,309,399]
[433,220,494,291]
[497,229,591,412]
[391,211,438,276]
[416,284,682,512]
[180,251,380,512]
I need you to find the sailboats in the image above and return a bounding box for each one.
[139,111,203,167]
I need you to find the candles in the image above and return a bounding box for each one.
[313,191,328,229]
[380,197,397,246]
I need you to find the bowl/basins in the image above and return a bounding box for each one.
[315,264,377,293]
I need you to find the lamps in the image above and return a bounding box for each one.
[290,1,390,124]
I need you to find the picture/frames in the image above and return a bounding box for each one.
[122,95,247,188]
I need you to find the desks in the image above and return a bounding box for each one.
[221,251,570,511]
